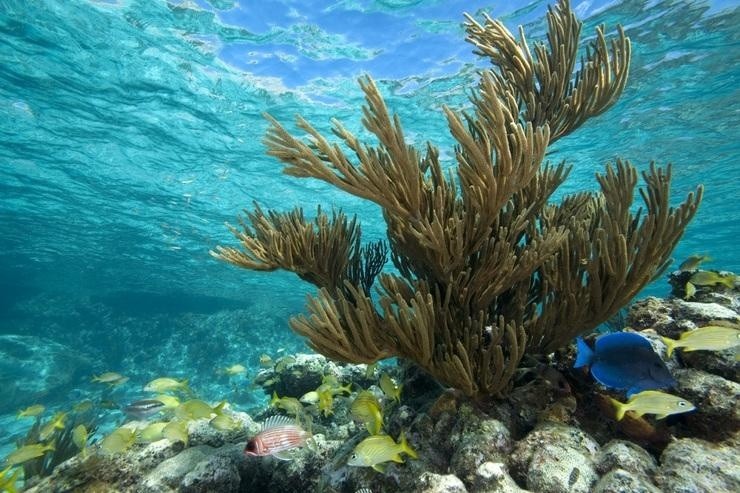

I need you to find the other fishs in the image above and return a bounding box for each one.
[225,363,247,376]
[245,352,415,474]
[0,370,241,466]
